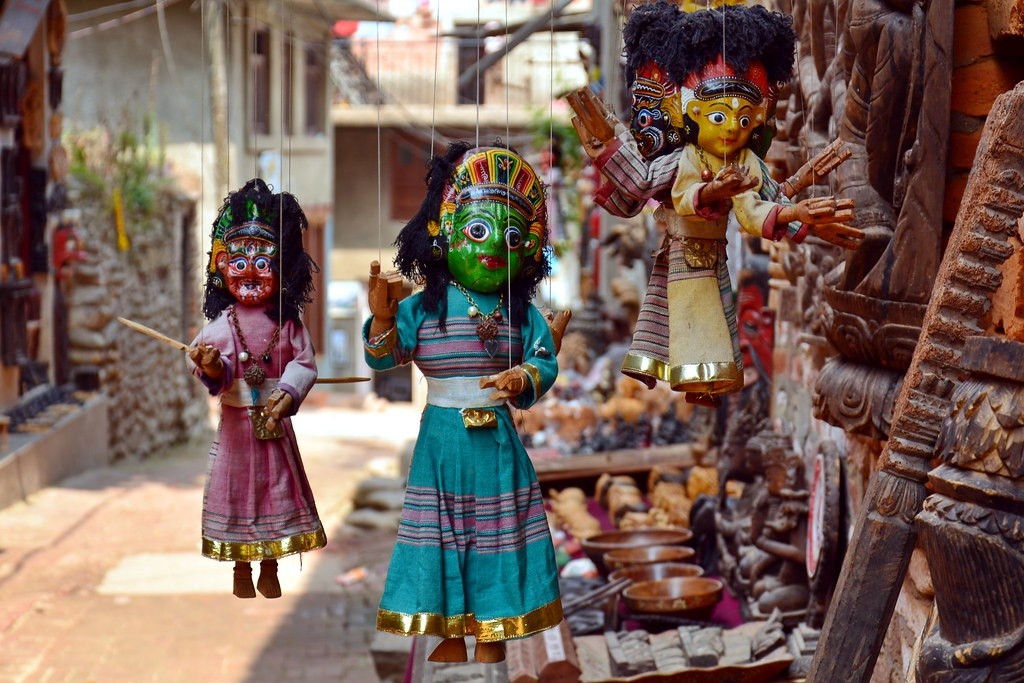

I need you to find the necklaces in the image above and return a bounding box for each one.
[447,280,505,359]
[695,144,740,212]
[229,302,285,385]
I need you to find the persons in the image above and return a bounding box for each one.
[114,178,327,599]
[564,0,867,408]
[362,141,567,664]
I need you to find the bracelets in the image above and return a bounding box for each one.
[785,175,803,196]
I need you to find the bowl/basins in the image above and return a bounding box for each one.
[582,528,705,596]
[622,576,724,633]
